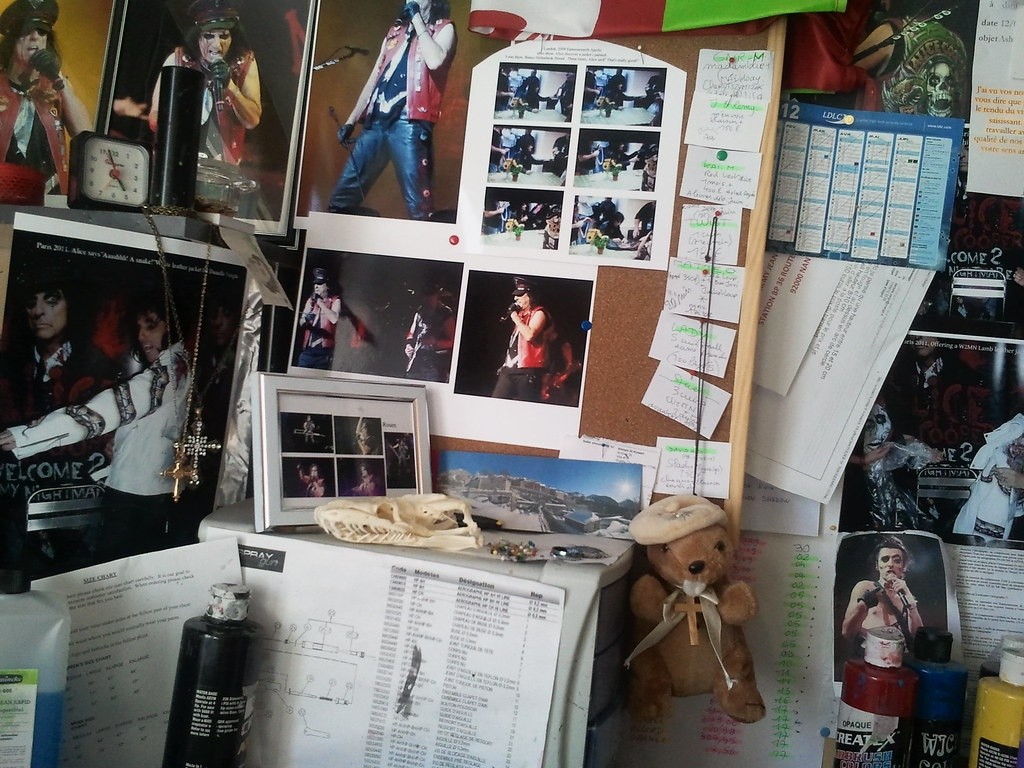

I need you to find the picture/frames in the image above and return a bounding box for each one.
[93,0,322,237]
[0,211,248,594]
[250,372,433,534]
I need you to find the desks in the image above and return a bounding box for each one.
[197,492,636,768]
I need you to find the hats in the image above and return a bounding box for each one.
[313,268,335,285]
[512,277,539,296]
[0,1,59,39]
[187,0,240,30]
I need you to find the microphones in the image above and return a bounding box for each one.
[210,56,224,111]
[500,302,518,322]
[345,46,370,55]
[311,300,316,311]
[43,65,66,90]
[395,8,410,27]
[888,572,912,608]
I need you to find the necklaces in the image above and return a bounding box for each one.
[141,201,222,502]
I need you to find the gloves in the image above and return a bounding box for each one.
[404,1,421,24]
[508,303,517,316]
[29,52,59,81]
[310,292,321,304]
[210,59,232,88]
[338,124,356,148]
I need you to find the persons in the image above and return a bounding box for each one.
[482,60,665,263]
[328,0,459,220]
[847,335,1024,544]
[147,1,264,169]
[1013,267,1024,286]
[299,267,341,369]
[842,535,923,652]
[489,274,574,402]
[296,416,412,496]
[0,0,94,206]
[404,286,455,383]
[1,269,237,566]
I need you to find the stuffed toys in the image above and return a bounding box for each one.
[615,493,768,724]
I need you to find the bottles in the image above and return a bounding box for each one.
[161,581,261,768]
[0,561,72,768]
[833,628,920,768]
[968,648,1024,768]
[979,635,1024,677]
[904,626,969,768]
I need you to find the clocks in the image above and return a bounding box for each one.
[67,131,156,213]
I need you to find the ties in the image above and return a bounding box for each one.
[916,374,926,408]
